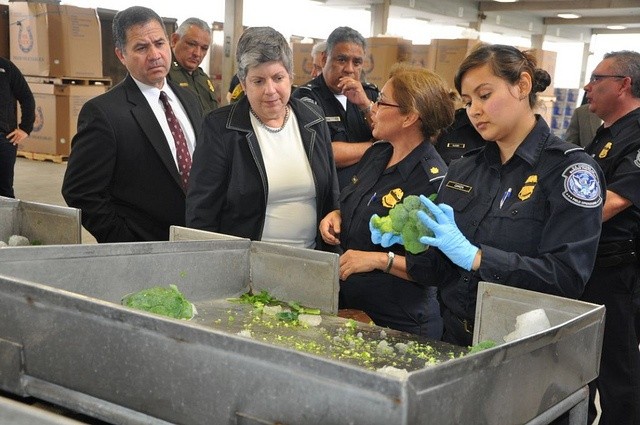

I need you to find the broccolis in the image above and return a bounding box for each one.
[369,194,437,254]
[122,283,193,320]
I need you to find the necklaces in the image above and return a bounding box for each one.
[250,105,289,132]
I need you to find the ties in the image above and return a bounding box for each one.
[159,93,192,193]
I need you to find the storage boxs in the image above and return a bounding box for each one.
[429,39,489,90]
[533,97,556,130]
[515,46,557,97]
[414,45,430,69]
[365,38,412,92]
[291,35,324,87]
[9,0,102,77]
[210,22,223,77]
[98,6,176,85]
[16,83,109,155]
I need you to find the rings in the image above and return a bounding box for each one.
[350,80,356,85]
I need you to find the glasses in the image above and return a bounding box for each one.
[590,74,625,84]
[375,96,400,108]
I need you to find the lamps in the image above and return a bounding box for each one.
[557,13,581,19]
[607,25,626,30]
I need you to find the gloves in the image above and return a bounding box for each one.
[369,214,405,247]
[417,193,478,271]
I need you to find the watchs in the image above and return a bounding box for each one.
[383,251,395,274]
[360,100,374,114]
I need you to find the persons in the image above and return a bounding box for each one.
[228,75,242,104]
[0,57,36,196]
[165,18,218,140]
[60,6,205,242]
[583,48,640,425]
[436,108,487,166]
[292,26,380,191]
[368,44,607,346]
[563,103,605,149]
[318,62,455,339]
[185,26,340,252]
[309,41,327,78]
